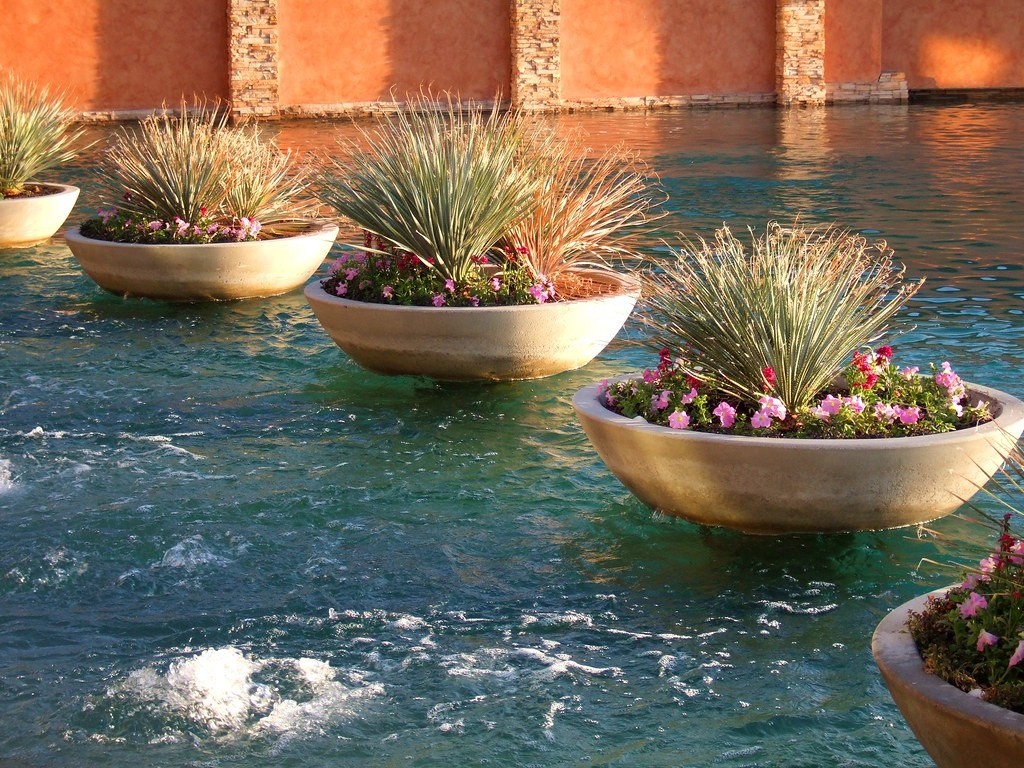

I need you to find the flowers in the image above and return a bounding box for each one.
[71,87,344,241]
[922,418,1024,716]
[300,79,679,307]
[597,210,995,440]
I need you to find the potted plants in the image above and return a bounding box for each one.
[0,66,106,249]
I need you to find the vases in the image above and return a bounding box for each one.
[874,582,1024,768]
[570,370,1024,535]
[62,223,340,302]
[299,263,643,380]
